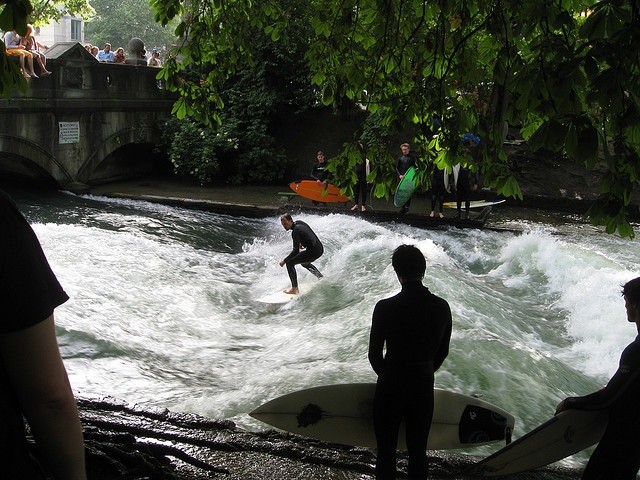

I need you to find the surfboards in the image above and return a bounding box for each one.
[287,179,354,204]
[393,165,422,208]
[462,387,614,477]
[452,162,460,191]
[248,382,515,450]
[444,167,452,193]
[443,200,487,206]
[256,282,314,304]
[366,158,370,182]
[443,200,506,208]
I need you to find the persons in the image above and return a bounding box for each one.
[0,191,88,480]
[368,244,452,480]
[279,213,324,294]
[554,277,640,480]
[456,164,478,219]
[396,143,415,213]
[310,150,333,206]
[350,141,372,211]
[96,43,114,63]
[4,29,39,79]
[430,163,453,218]
[114,47,125,64]
[19,24,52,76]
[84,44,99,62]
[143,50,161,67]
